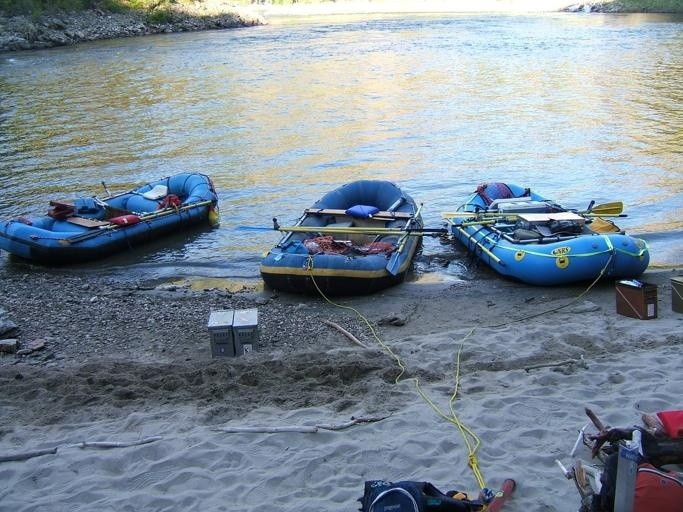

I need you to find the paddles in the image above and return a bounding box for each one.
[234,226,447,237]
[66,197,218,245]
[441,212,507,268]
[450,200,622,227]
[442,211,628,220]
[386,202,423,277]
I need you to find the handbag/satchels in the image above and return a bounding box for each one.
[549,218,583,234]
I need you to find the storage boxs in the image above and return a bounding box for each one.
[207,308,262,359]
[616,275,683,320]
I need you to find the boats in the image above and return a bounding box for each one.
[0,170,221,264]
[451,183,650,289]
[259,178,425,293]
